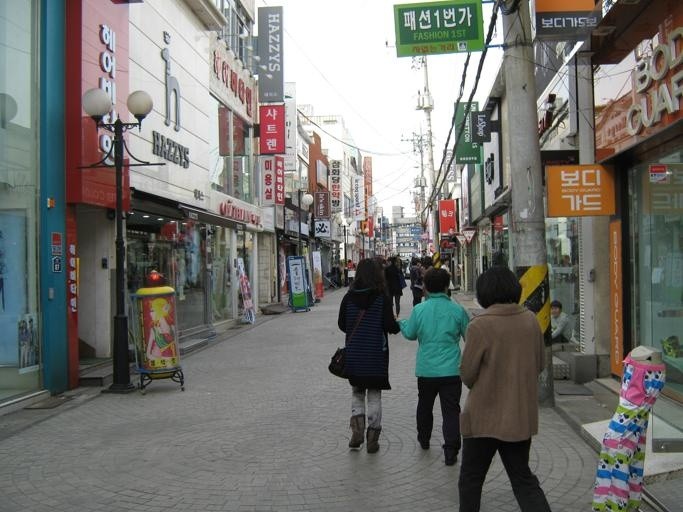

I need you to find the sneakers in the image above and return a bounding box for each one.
[417,433,431,450]
[444,449,457,466]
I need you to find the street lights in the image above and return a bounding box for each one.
[335,214,389,288]
[296,189,313,256]
[0,90,20,131]
[73,83,170,396]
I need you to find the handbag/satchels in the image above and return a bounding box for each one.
[399,273,407,288]
[328,348,350,379]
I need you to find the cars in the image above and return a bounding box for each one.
[399,256,411,279]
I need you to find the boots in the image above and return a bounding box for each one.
[348,414,365,448]
[367,426,382,454]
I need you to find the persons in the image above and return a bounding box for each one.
[458,266,552,512]
[19,319,37,369]
[551,300,572,343]
[338,258,470,466]
